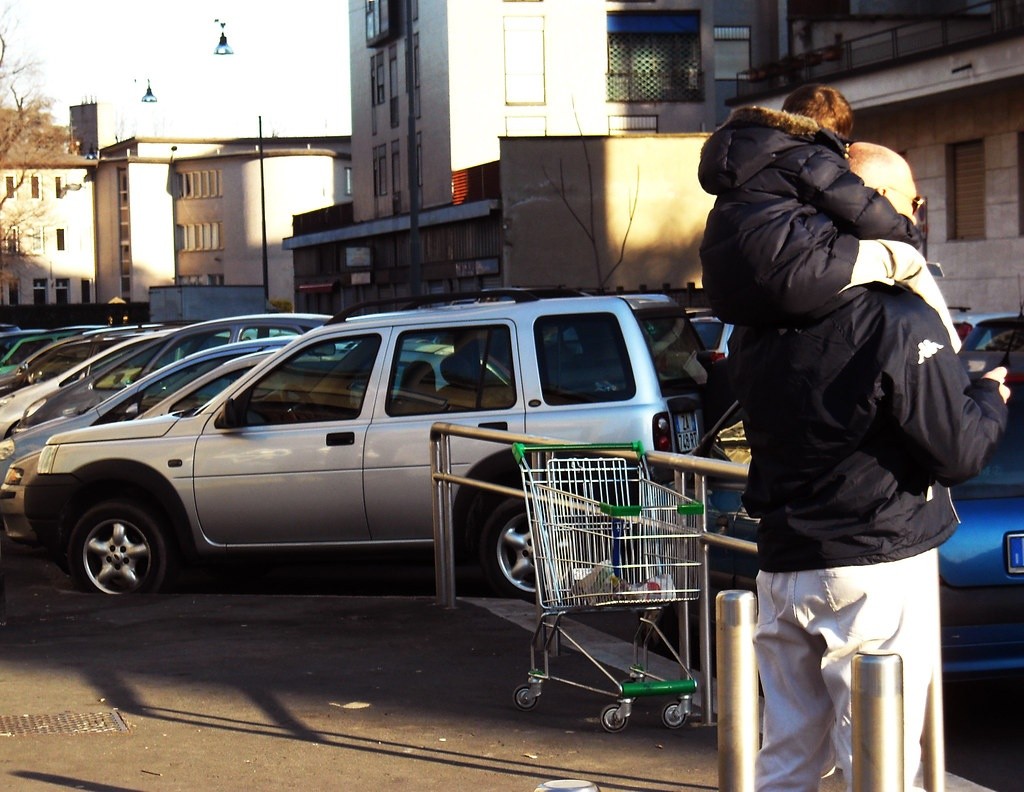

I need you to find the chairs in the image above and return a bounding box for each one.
[436,355,474,412]
[392,360,435,413]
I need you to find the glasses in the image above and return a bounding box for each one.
[883,185,925,215]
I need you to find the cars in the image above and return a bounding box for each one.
[0,317,523,544]
[668,304,1024,735]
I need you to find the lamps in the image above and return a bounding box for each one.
[213,23,234,55]
[142,79,157,102]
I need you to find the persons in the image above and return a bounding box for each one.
[718,139,1011,792]
[699,84,964,362]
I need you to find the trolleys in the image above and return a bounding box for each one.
[508,442,706,728]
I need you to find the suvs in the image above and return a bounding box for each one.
[30,294,727,596]
